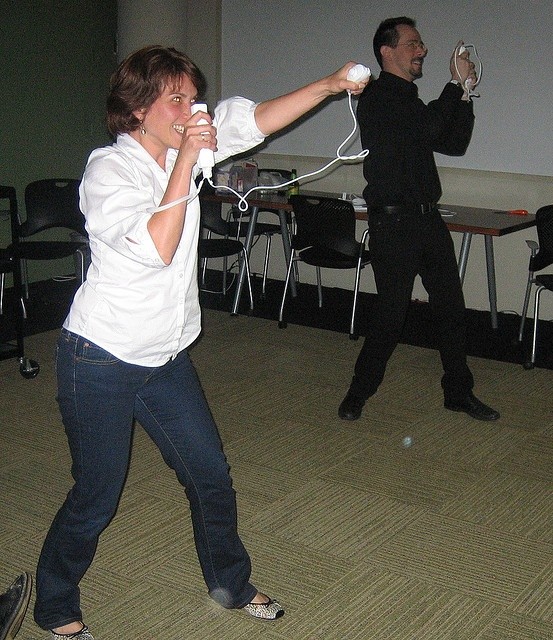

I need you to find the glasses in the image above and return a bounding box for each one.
[400,42,425,50]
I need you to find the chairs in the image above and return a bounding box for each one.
[1,184,41,379]
[197,235,256,312]
[509,205,553,371]
[1,177,93,316]
[194,166,301,300]
[277,194,372,342]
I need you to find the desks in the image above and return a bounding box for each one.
[200,189,537,360]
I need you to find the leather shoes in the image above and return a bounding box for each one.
[244,592,284,620]
[0,572,32,639]
[48,620,94,640]
[338,394,365,420]
[443,387,500,421]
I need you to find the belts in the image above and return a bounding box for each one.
[372,201,438,215]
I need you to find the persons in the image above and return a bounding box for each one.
[29,41,372,640]
[334,15,501,422]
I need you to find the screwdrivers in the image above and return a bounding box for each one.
[493,209,529,215]
